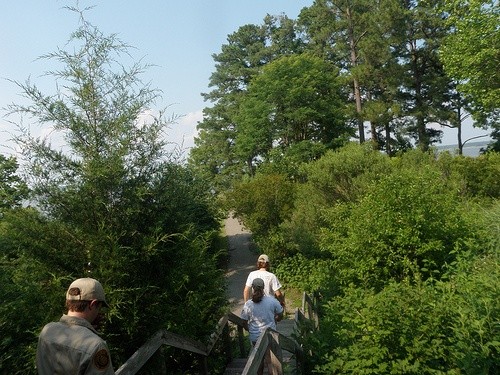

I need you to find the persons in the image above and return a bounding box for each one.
[240,278,283,346]
[243,254,285,302]
[36,277,115,375]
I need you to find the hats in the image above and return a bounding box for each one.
[252,278,264,290]
[65,277,110,309]
[258,254,269,263]
